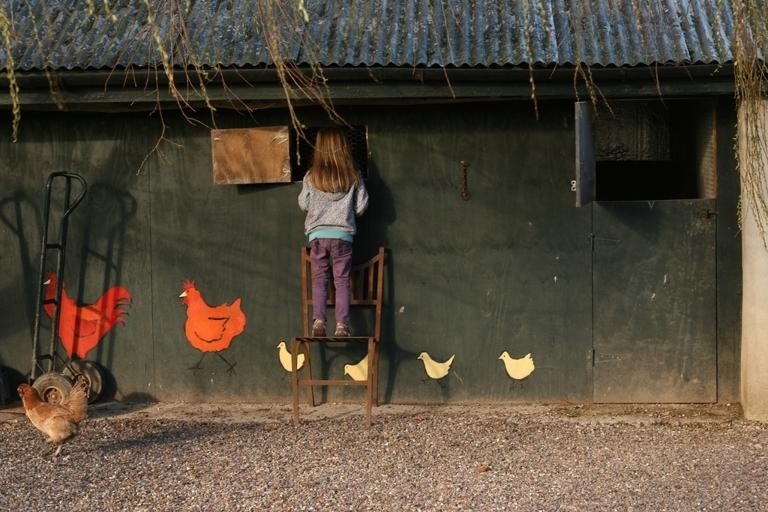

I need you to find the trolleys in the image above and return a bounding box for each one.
[26,171,104,408]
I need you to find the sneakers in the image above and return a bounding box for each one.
[334,323,352,337]
[313,319,327,337]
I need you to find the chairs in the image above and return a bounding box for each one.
[289,246,385,419]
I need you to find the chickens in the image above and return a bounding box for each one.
[16,379,89,457]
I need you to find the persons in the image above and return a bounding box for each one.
[298,127,369,337]
